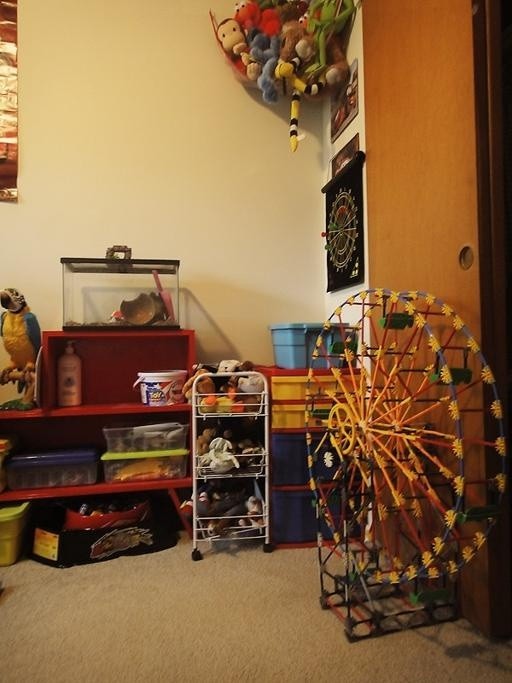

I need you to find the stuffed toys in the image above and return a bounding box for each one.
[216,0,355,152]
[181,357,266,537]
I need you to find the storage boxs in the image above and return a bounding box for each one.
[102,421,189,452]
[6,439,100,489]
[2,499,31,566]
[99,447,189,483]
[270,321,349,369]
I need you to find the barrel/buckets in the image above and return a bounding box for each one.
[132,369,188,407]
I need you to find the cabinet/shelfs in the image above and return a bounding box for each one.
[1,330,197,535]
[258,363,365,547]
[191,371,273,560]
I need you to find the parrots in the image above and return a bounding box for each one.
[0,287,41,405]
